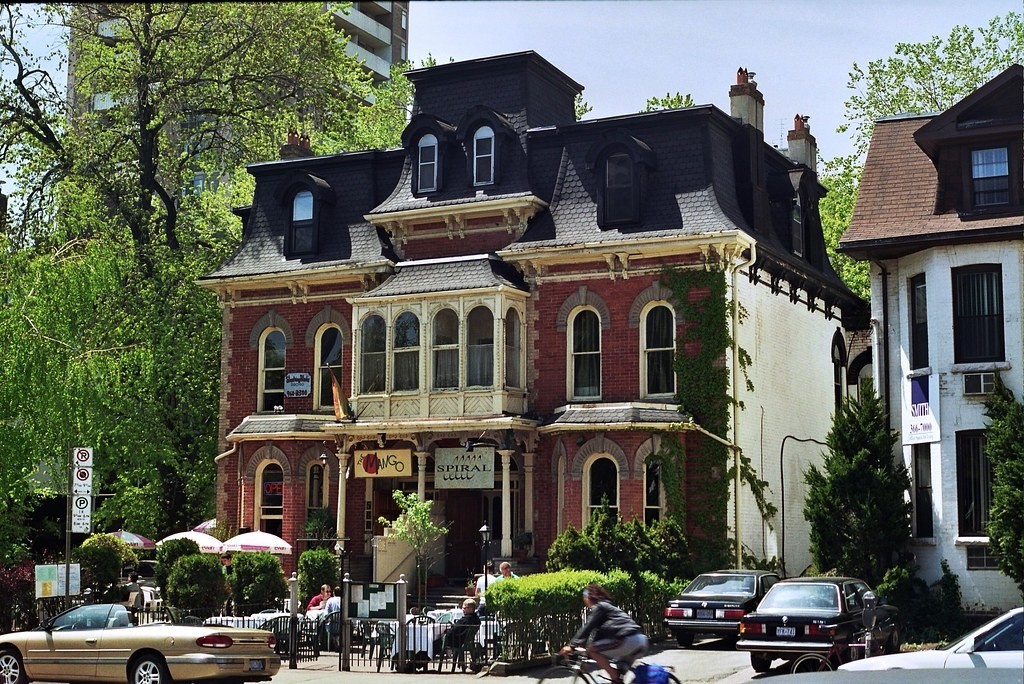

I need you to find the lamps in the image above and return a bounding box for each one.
[575,437,587,447]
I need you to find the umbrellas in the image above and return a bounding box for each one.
[155,518,223,554]
[223,530,292,555]
[112,531,155,582]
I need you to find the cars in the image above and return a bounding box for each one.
[736,577,903,671]
[837,606,1024,671]
[0,603,281,684]
[662,570,782,647]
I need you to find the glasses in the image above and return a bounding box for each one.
[326,590,332,593]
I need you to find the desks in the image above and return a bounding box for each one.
[205,616,260,628]
[252,612,303,638]
[390,624,451,671]
[306,610,327,647]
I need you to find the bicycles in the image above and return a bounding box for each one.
[538,647,682,684]
[791,618,895,675]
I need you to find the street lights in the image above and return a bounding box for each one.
[479,521,491,666]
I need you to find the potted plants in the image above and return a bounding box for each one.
[930,558,964,627]
[416,567,430,594]
[465,578,475,596]
[513,533,531,563]
[427,571,442,587]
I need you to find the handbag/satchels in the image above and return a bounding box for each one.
[636,665,669,684]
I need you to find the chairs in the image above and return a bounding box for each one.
[140,585,455,672]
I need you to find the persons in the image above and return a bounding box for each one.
[307,584,331,610]
[127,574,139,591]
[416,598,481,669]
[475,562,497,616]
[496,562,518,582]
[325,586,341,631]
[221,554,233,575]
[560,585,648,684]
